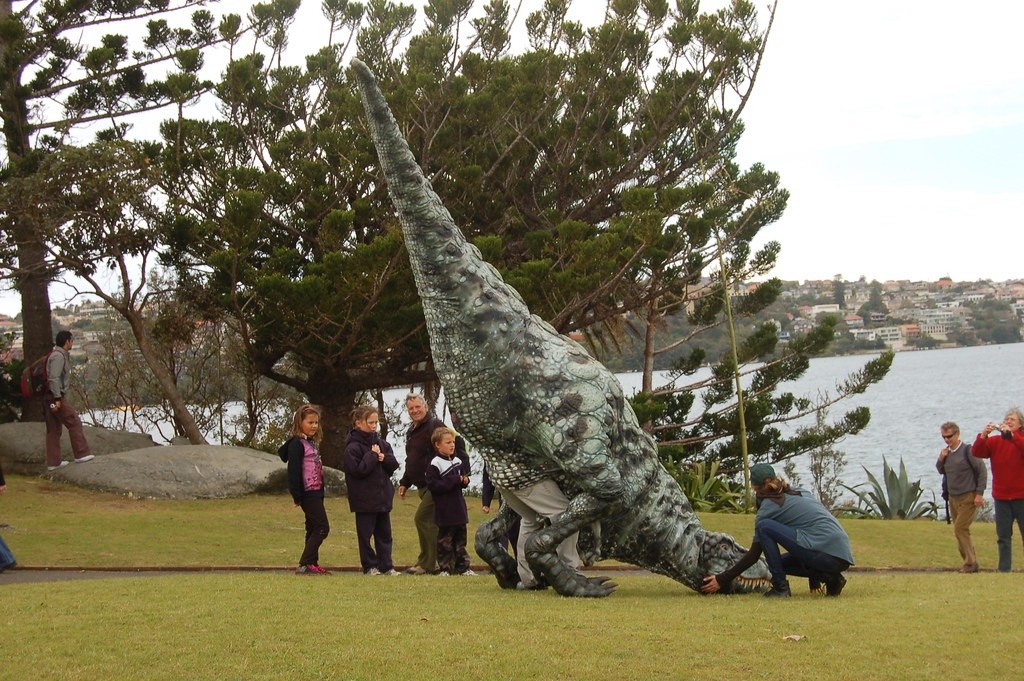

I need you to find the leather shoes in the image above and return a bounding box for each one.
[516,576,551,589]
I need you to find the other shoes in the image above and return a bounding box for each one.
[964,562,979,572]
[0,560,16,574]
[48,461,69,471]
[75,454,94,463]
[482,505,491,513]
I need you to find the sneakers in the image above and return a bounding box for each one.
[439,569,479,576]
[407,560,441,576]
[767,580,791,597]
[295,563,332,576]
[826,572,847,596]
[367,568,402,576]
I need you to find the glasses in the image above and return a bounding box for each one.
[942,430,957,438]
[1004,418,1020,422]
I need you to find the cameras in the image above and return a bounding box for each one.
[990,424,1003,432]
[48,401,56,409]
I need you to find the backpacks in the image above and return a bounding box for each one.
[22,350,66,405]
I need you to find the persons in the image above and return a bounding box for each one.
[936,422,987,573]
[481,459,588,590]
[343,405,401,575]
[971,409,1024,572]
[398,394,471,574]
[278,405,331,575]
[704,463,854,597]
[425,428,477,576]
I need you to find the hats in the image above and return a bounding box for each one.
[750,463,775,486]
